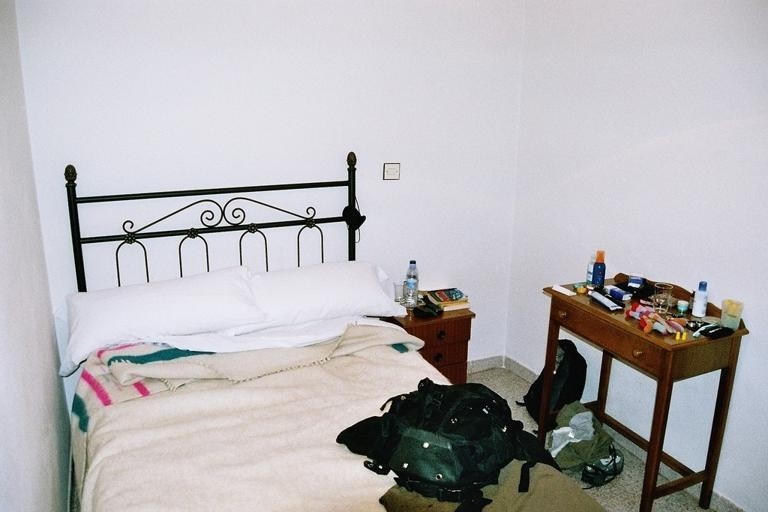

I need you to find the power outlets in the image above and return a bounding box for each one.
[383,163,399,180]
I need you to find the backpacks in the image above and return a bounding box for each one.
[336,375,520,500]
[522,341,588,424]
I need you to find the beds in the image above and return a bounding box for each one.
[64,152,609,512]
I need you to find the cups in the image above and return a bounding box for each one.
[393,281,404,302]
[720,299,744,331]
[654,283,673,314]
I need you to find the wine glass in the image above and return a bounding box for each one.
[677,300,688,315]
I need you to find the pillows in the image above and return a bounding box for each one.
[59,266,264,376]
[236,261,407,339]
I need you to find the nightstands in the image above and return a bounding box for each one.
[396,303,475,385]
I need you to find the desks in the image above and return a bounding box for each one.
[538,278,750,511]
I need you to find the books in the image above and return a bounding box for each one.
[427,287,471,312]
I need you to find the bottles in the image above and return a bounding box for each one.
[689,281,709,317]
[402,260,418,310]
[586,250,606,288]
